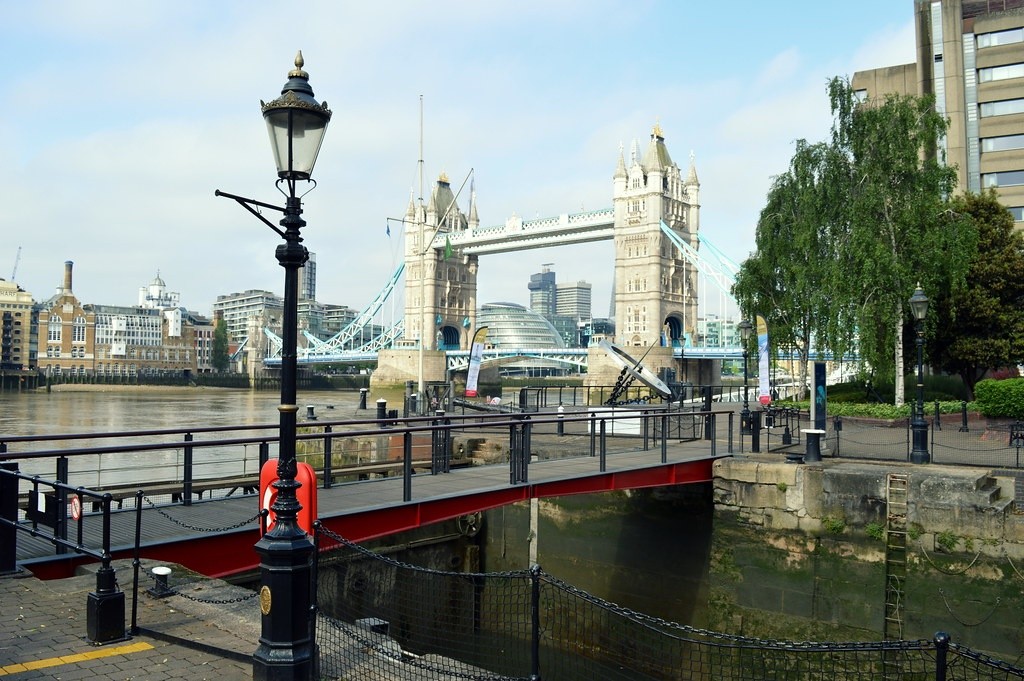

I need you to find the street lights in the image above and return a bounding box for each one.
[736,318,752,436]
[678,334,686,402]
[907,280,931,464]
[214,49,333,681]
